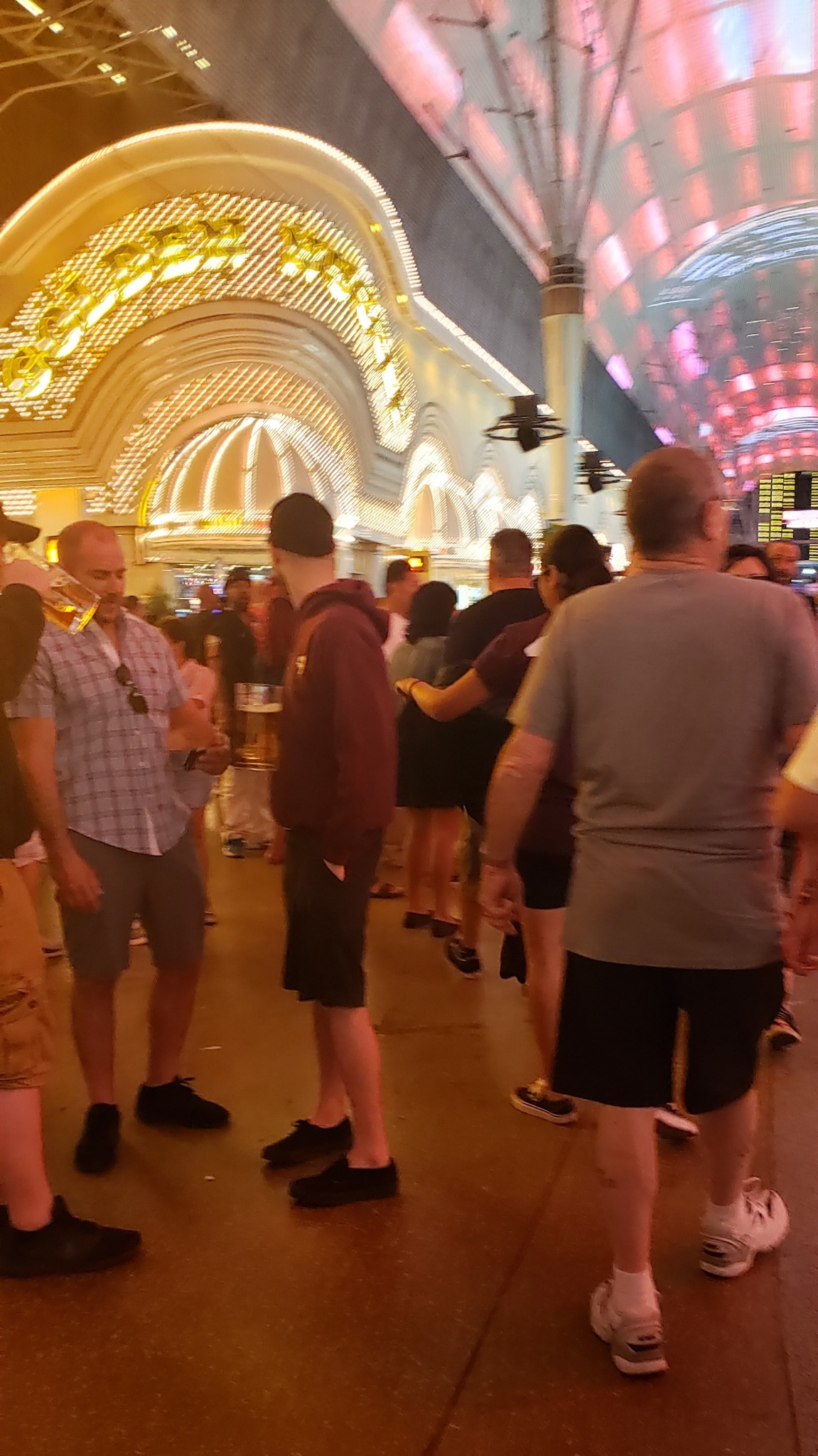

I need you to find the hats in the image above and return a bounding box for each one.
[224,569,250,589]
[271,494,335,556]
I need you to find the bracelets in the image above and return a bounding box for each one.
[408,679,426,696]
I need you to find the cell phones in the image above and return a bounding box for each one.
[184,747,206,770]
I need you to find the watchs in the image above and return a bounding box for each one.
[476,845,514,866]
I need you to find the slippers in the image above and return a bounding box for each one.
[371,880,404,898]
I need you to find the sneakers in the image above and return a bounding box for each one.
[1,1195,141,1277]
[134,1076,231,1129]
[654,1103,699,1138]
[222,840,245,857]
[128,918,149,946]
[42,946,66,957]
[261,1114,353,1163]
[403,910,437,928]
[763,1006,802,1050]
[288,1155,398,1206]
[590,1278,667,1373]
[443,938,482,977]
[430,916,462,937]
[698,1190,788,1276]
[73,1102,121,1174]
[510,1085,577,1124]
[202,910,218,927]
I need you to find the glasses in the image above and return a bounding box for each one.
[116,663,150,715]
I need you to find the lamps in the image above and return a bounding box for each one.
[480,390,621,495]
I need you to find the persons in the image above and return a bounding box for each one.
[1,491,818,1279]
[479,446,818,1379]
